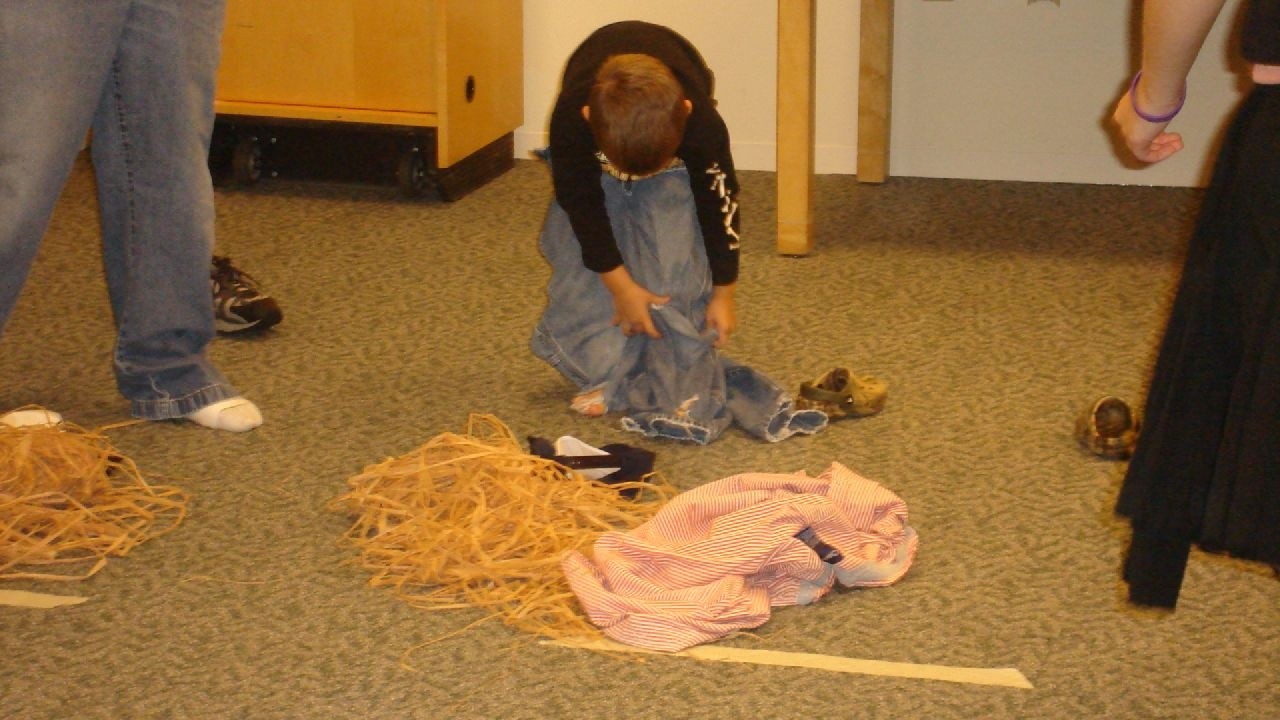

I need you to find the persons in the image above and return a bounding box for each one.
[529,20,740,415]
[1100,0,1280,614]
[0,0,263,433]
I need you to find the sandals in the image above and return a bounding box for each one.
[1076,397,1138,458]
[794,366,887,418]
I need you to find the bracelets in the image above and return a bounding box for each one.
[1131,70,1187,123]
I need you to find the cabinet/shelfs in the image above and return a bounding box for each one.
[205,0,527,205]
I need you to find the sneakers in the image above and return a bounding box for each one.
[206,256,283,334]
